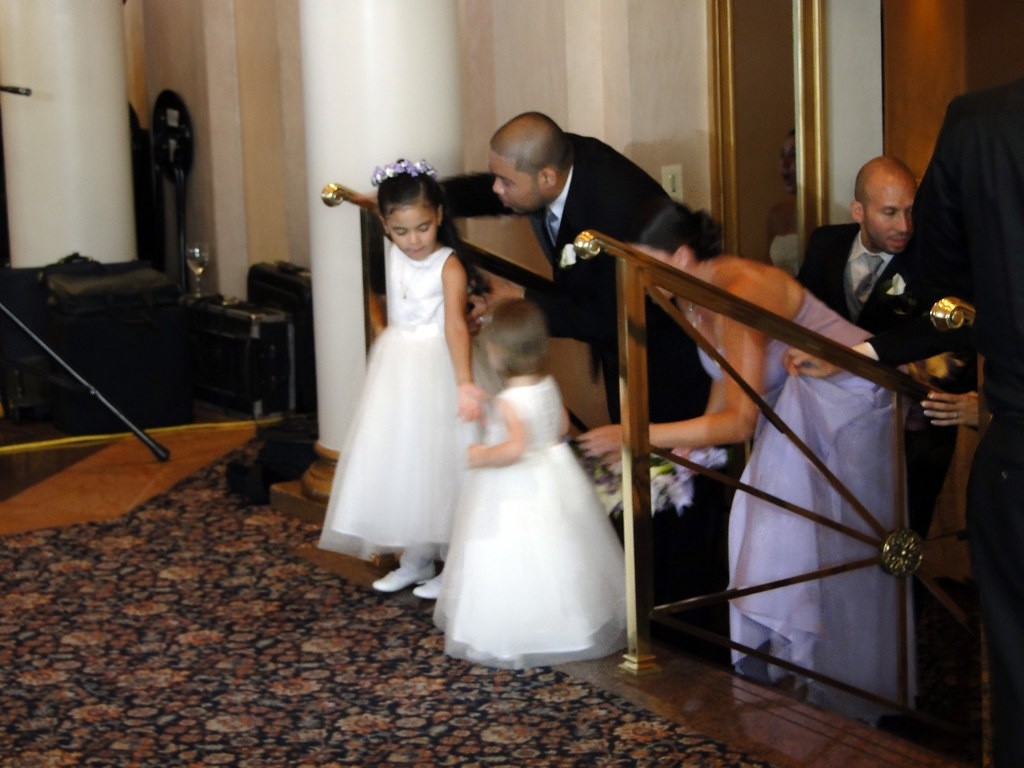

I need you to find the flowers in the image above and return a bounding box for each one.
[569,436,730,518]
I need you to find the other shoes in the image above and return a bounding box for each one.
[371,567,436,592]
[411,577,439,599]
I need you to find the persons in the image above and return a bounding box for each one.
[317,158,506,599]
[782,76,1024,768]
[370,110,746,670]
[575,201,921,720]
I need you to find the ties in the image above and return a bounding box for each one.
[545,210,556,250]
[852,256,883,304]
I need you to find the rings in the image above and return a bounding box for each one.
[953,412,957,419]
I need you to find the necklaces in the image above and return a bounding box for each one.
[688,260,702,327]
[398,255,428,298]
